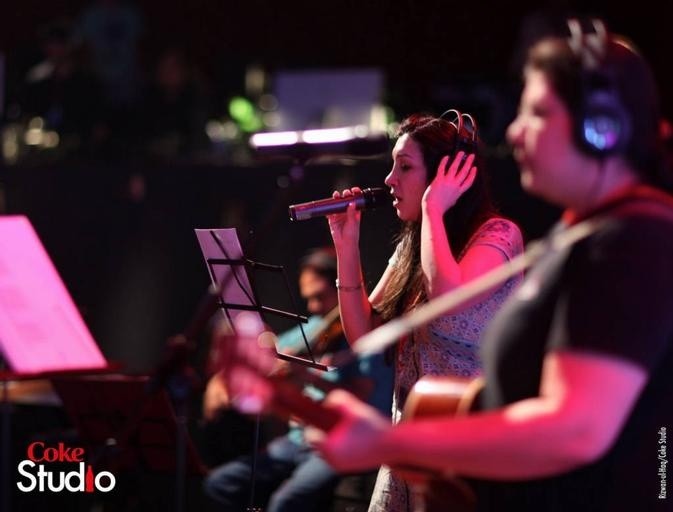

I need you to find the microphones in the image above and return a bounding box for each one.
[291,187,388,219]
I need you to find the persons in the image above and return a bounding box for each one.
[327,111,523,512]
[204,249,387,512]
[304,31,673,512]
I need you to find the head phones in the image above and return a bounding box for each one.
[566,20,631,155]
[441,109,484,174]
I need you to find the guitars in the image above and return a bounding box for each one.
[210,321,489,512]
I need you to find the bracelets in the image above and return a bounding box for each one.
[335,281,364,293]
